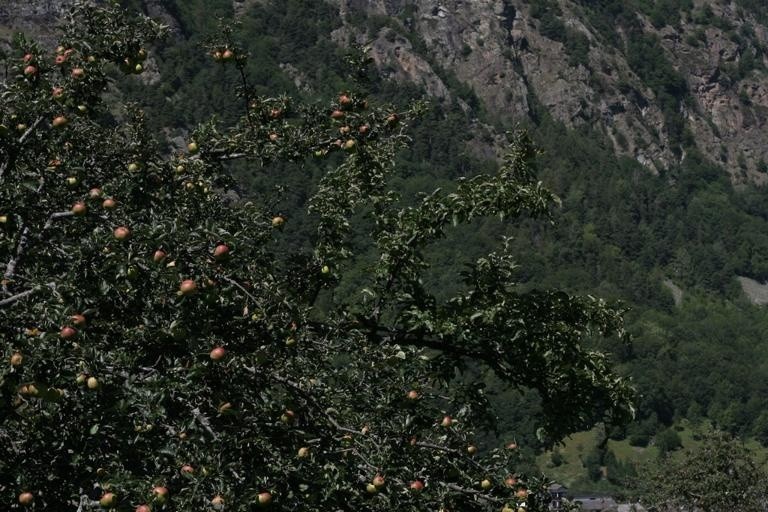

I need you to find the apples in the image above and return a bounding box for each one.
[0,1,529,512]
